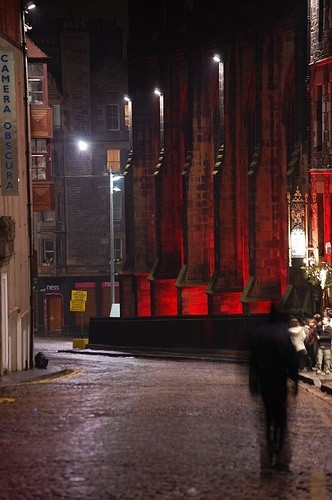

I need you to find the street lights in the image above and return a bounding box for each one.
[77,138,116,306]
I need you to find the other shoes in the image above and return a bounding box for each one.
[297,366,332,376]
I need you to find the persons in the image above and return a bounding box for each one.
[289,304,332,375]
[249,313,299,478]
